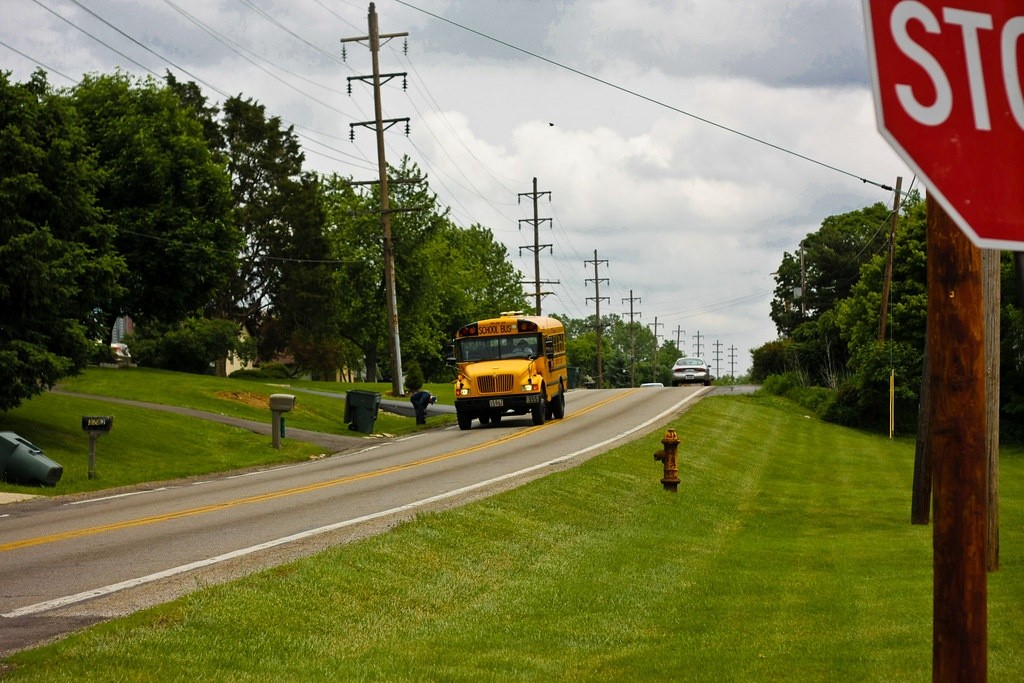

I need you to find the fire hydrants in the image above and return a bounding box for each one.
[653,427,681,492]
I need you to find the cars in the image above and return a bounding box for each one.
[86,339,131,366]
[669,356,712,387]
[640,382,664,388]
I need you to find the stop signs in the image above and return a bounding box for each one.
[864,0,1024,253]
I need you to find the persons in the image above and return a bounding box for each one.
[512,339,532,353]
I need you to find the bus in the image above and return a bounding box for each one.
[446,311,568,430]
